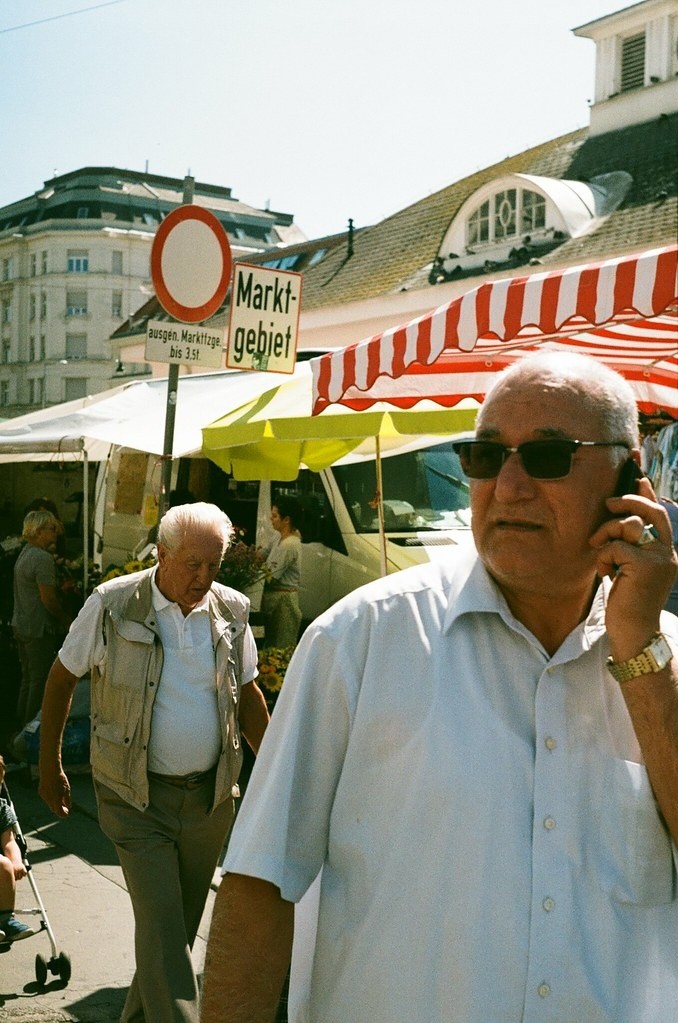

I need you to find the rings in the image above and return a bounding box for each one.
[637,524,660,543]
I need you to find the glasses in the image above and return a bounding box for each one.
[40,526,56,532]
[453,440,630,479]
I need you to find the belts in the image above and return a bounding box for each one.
[147,769,217,790]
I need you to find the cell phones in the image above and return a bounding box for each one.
[608,457,654,570]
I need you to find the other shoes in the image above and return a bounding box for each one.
[0,916,34,940]
[0,929,5,941]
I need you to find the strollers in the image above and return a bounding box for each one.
[0,777,72,996]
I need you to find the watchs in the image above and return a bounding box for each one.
[606,631,674,683]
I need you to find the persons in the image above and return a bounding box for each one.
[39,502,269,1023]
[5,499,73,728]
[256,497,302,653]
[146,489,199,546]
[0,756,34,950]
[199,348,678,1023]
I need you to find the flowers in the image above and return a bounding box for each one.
[217,526,287,590]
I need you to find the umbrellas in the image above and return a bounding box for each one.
[204,368,482,577]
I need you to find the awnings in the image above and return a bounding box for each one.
[306,245,676,485]
[0,361,308,458]
